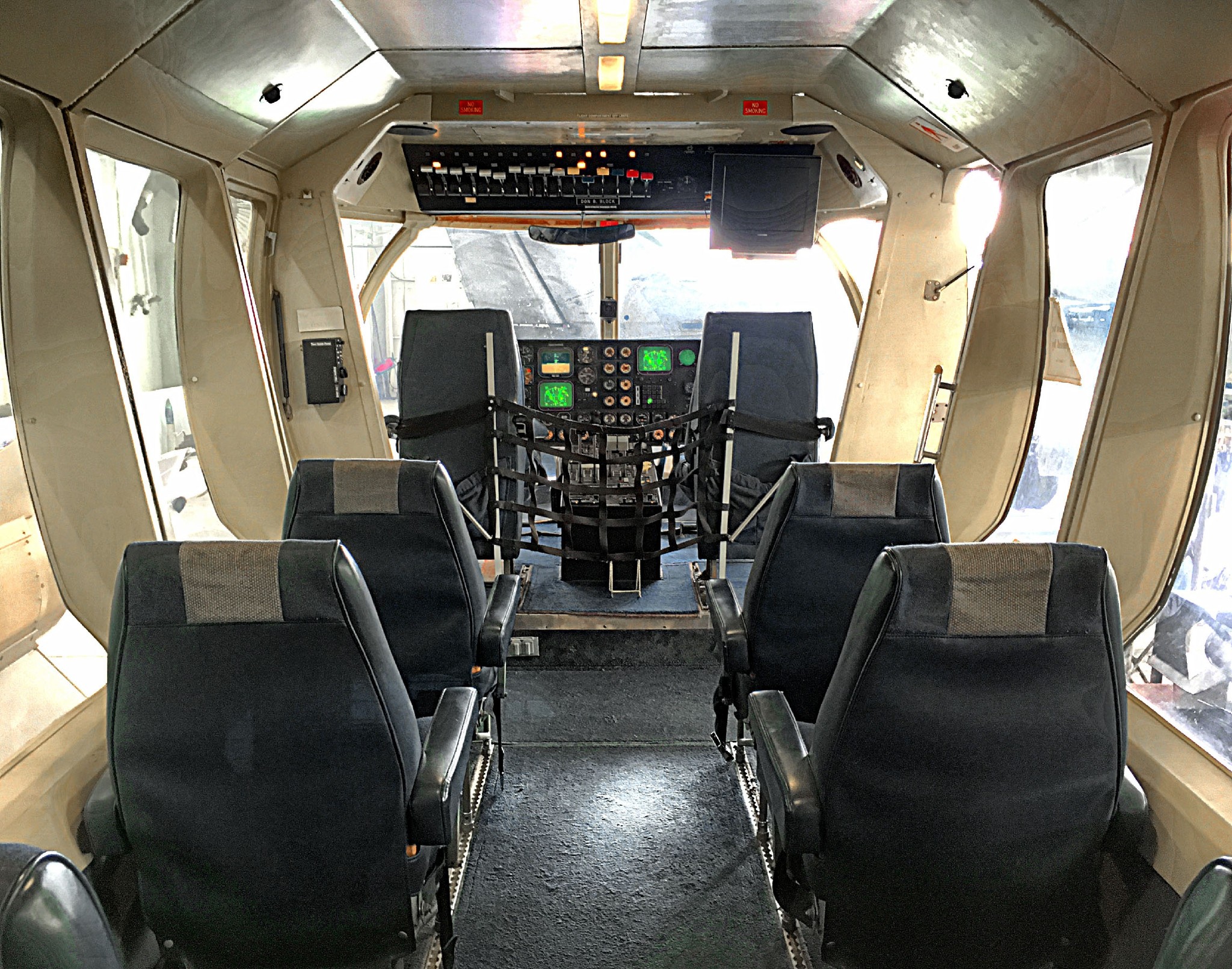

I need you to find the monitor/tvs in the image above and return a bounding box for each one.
[709,151,821,254]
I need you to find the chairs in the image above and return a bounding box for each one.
[668,309,1232,969]
[0,305,528,969]
